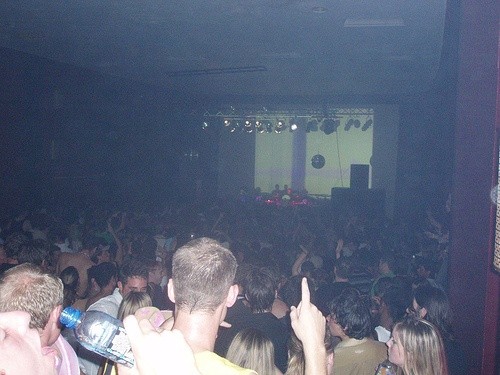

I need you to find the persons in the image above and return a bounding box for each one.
[0,262,83,374]
[119,236,329,375]
[0,178,450,331]
[0,311,61,374]
[281,326,334,375]
[72,264,149,375]
[324,290,391,375]
[377,315,448,375]
[226,327,285,375]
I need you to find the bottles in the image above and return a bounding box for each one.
[375,359,392,375]
[59,306,137,368]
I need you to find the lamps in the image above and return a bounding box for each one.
[223,115,372,134]
